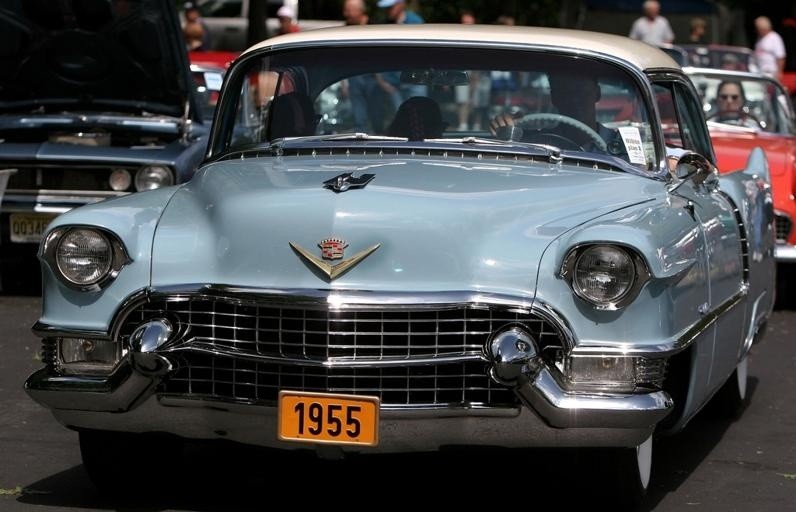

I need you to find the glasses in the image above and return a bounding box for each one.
[720,95,737,99]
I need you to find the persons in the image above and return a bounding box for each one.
[490,16,518,90]
[181,7,205,52]
[274,6,301,35]
[628,0,787,131]
[488,72,616,151]
[454,12,483,131]
[375,0,429,117]
[334,0,387,134]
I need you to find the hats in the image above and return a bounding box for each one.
[377,0,405,7]
[278,7,294,17]
